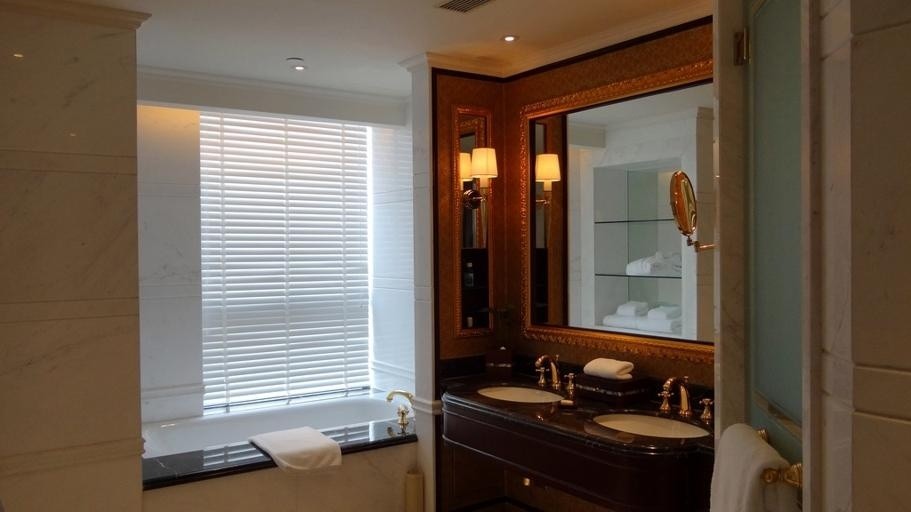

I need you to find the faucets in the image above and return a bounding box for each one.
[387,390,415,408]
[664,376,692,415]
[536,353,559,384]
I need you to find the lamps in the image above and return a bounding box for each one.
[531,153,563,210]
[464,149,499,210]
[458,152,472,211]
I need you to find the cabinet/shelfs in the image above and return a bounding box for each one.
[594,216,683,279]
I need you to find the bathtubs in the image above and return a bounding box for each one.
[141,389,415,459]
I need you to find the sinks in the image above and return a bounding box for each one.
[478,384,565,404]
[592,410,710,441]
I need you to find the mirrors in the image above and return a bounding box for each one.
[449,101,496,341]
[670,168,713,251]
[518,61,714,366]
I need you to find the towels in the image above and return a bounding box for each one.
[623,250,683,279]
[601,299,681,334]
[710,424,797,511]
[248,425,343,477]
[583,358,634,381]
